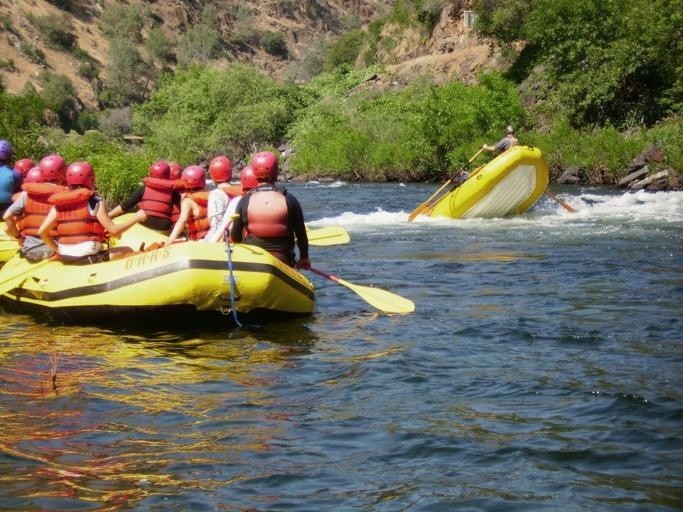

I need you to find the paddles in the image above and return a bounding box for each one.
[0,255,60,295]
[294,226,350,247]
[407,148,485,223]
[0,222,19,261]
[544,190,578,213]
[423,162,487,216]
[295,261,416,316]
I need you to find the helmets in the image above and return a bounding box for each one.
[240,166,258,189]
[168,163,182,179]
[181,166,206,189]
[506,126,515,134]
[15,159,34,177]
[0,140,12,160]
[150,161,169,179]
[26,167,43,183]
[40,155,66,184]
[210,157,232,182]
[252,152,278,179]
[66,162,95,190]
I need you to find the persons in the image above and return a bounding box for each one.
[38,162,147,265]
[210,167,259,242]
[227,152,312,271]
[166,162,183,225]
[0,140,22,222]
[108,161,181,231]
[204,156,236,241]
[3,155,67,261]
[484,125,518,152]
[165,165,212,245]
[13,158,36,178]
[449,164,467,191]
[12,168,42,200]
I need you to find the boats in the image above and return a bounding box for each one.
[419,145,549,219]
[0,240,316,317]
[110,213,188,251]
[0,223,19,262]
